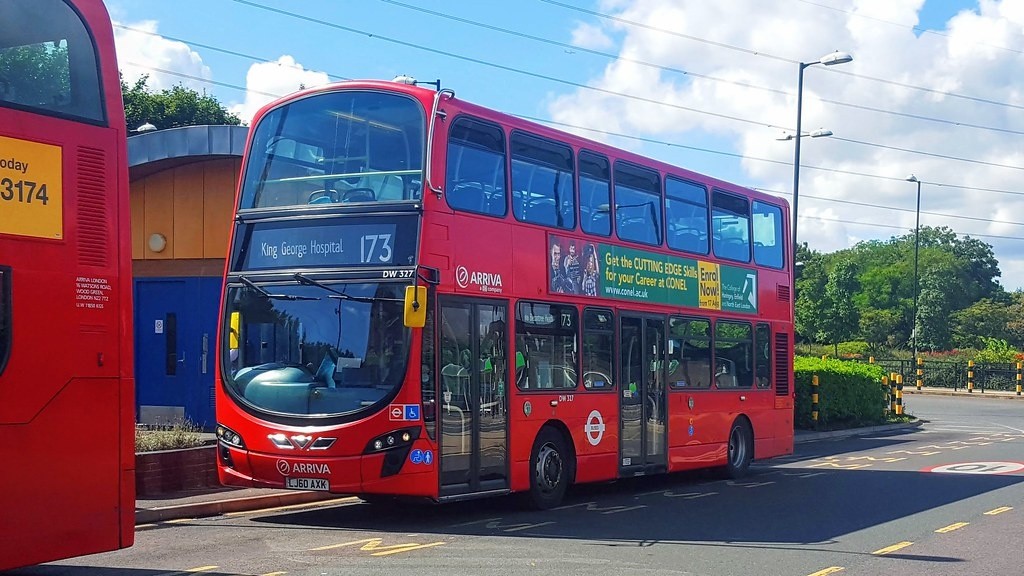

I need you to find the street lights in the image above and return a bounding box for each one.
[904,174,921,372]
[703,216,741,233]
[779,51,855,287]
[597,202,661,247]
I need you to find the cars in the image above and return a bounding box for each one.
[516,362,613,389]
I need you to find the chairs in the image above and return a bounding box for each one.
[524,196,559,225]
[489,188,525,219]
[342,187,377,202]
[560,203,594,233]
[307,189,340,204]
[448,178,486,213]
[675,227,701,252]
[592,208,621,236]
[701,356,739,387]
[622,214,651,242]
[744,242,766,265]
[723,238,745,262]
[700,232,725,257]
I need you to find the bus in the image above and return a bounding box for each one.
[215,81,795,514]
[0,0,136,573]
